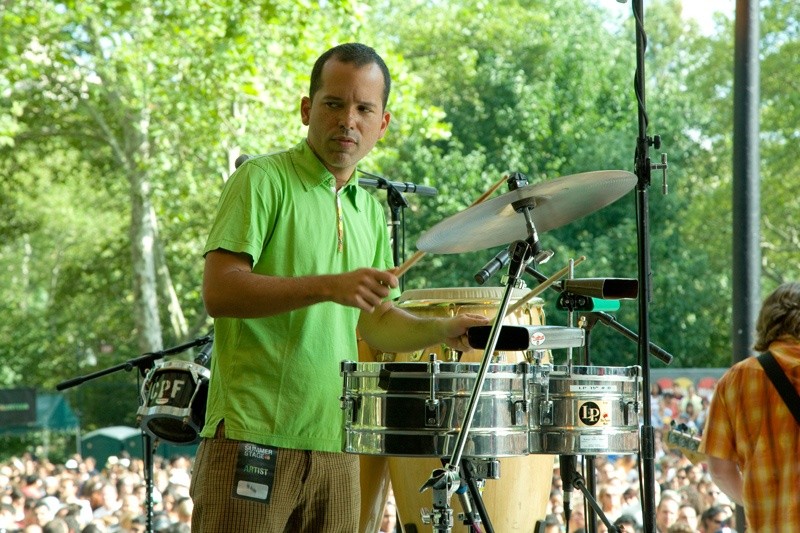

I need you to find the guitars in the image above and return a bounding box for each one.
[660,417,709,464]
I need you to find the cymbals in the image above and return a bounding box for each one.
[412,168,642,254]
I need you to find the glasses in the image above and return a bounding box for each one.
[708,517,724,524]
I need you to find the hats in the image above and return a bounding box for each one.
[64,459,78,471]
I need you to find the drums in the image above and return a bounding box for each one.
[142,360,214,448]
[547,361,646,458]
[341,356,549,460]
[386,285,554,533]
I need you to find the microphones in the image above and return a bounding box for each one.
[559,279,639,299]
[475,246,512,284]
[234,155,259,169]
[456,478,475,524]
[194,335,214,366]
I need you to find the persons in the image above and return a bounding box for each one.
[544,383,736,533]
[190,42,489,533]
[379,502,396,533]
[0,452,194,533]
[698,283,800,533]
